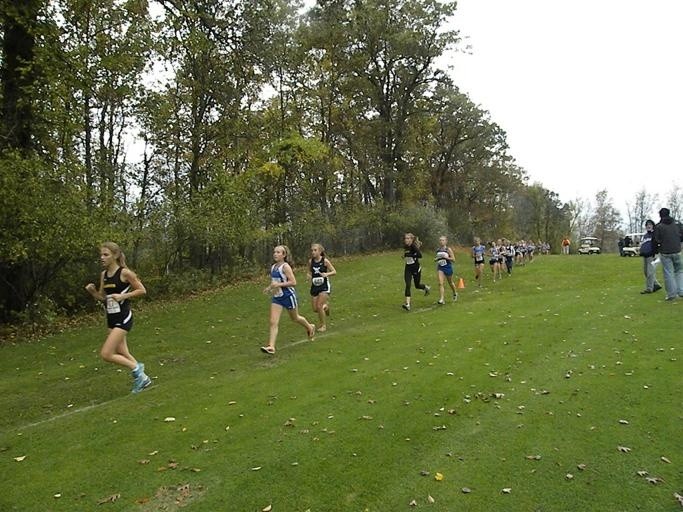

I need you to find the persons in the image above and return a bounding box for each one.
[85,242,151,394]
[488,238,550,283]
[654,208,683,301]
[401,233,431,311]
[471,238,485,287]
[562,239,570,255]
[639,220,662,294]
[305,244,336,332]
[619,236,633,257]
[260,245,315,355]
[434,235,458,305]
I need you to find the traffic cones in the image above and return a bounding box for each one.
[456,278,466,290]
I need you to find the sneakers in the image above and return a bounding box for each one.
[438,300,445,305]
[424,287,431,296]
[325,304,330,316]
[261,345,275,354]
[453,294,457,301]
[318,325,326,331]
[640,283,661,294]
[132,364,151,393]
[402,304,410,311]
[308,324,315,337]
